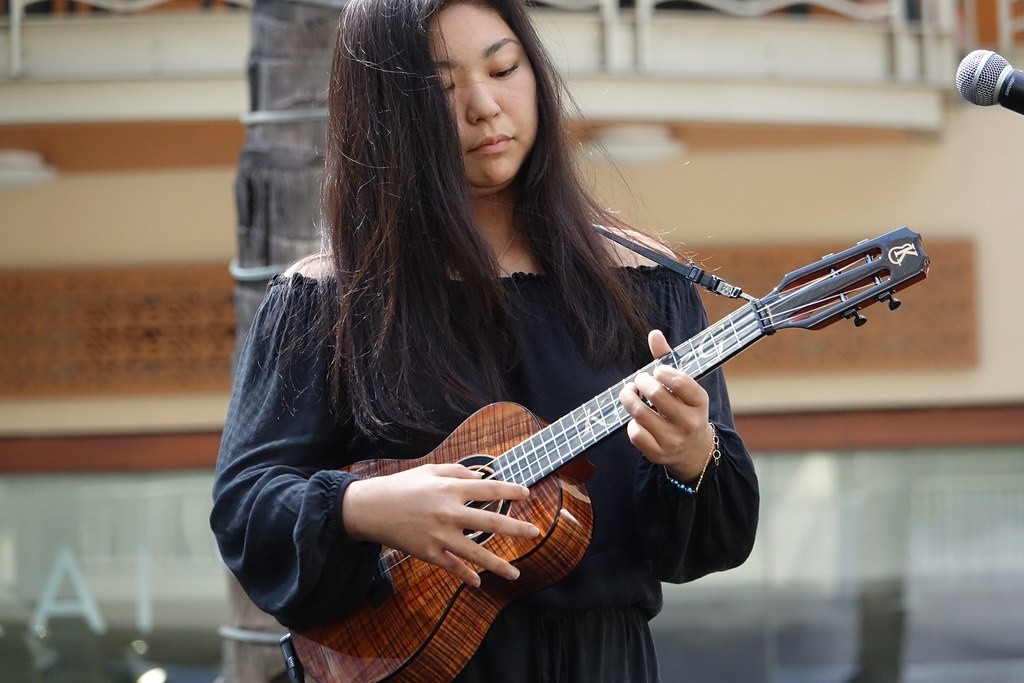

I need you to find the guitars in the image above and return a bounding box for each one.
[292,226,935,683]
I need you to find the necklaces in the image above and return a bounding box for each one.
[496,233,516,262]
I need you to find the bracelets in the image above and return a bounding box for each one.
[663,422,720,493]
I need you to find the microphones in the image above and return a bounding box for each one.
[956,50,1024,115]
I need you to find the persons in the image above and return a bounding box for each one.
[210,0,759,683]
